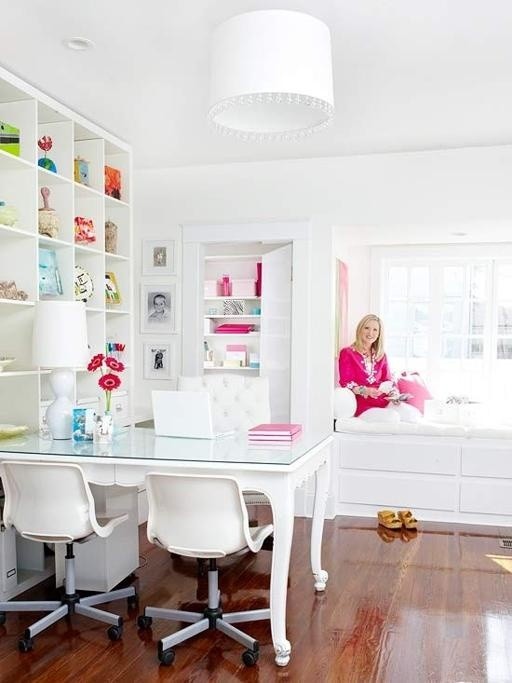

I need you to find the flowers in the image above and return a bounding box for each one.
[87,343,126,411]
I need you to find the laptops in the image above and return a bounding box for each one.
[151,389,237,440]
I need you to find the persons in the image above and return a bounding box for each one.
[338,312,392,419]
[148,293,169,322]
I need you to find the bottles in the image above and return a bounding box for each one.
[222,275,230,296]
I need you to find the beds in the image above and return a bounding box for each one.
[334,368,511,529]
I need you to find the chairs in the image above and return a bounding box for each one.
[170,375,273,562]
[0,460,139,653]
[137,472,273,666]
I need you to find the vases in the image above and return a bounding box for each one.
[104,410,118,443]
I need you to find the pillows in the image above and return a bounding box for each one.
[398,373,433,415]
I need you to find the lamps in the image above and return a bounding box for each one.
[205,10,336,144]
[31,301,88,440]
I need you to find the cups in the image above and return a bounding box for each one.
[73,408,95,440]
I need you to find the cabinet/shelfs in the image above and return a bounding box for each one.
[0,64,135,603]
[205,255,262,370]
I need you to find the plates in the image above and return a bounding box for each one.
[75,264,95,302]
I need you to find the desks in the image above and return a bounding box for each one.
[0,427,339,667]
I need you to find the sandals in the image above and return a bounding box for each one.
[378,510,402,529]
[398,510,418,529]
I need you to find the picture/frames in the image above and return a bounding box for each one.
[142,239,174,276]
[143,342,172,380]
[139,282,176,335]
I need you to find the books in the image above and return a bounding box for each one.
[248,430,302,440]
[248,444,291,450]
[248,440,298,445]
[248,423,302,434]
